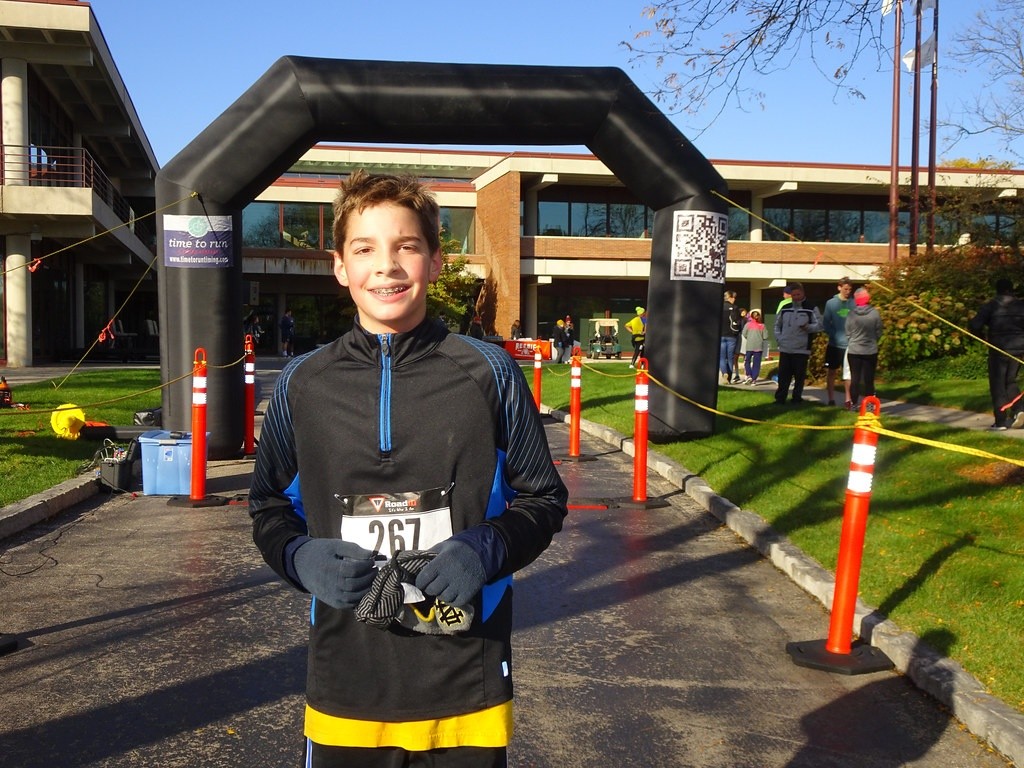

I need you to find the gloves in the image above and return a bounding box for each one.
[290,536,380,610]
[414,539,488,608]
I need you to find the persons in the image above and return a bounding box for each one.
[248,169,569,768]
[625,306,648,370]
[719,277,882,411]
[470,316,574,365]
[971,279,1024,430]
[249,307,296,359]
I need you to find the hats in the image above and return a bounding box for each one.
[565,315,571,322]
[994,278,1014,291]
[557,320,564,327]
[782,284,793,294]
[852,288,872,307]
[635,306,646,316]
[474,317,483,321]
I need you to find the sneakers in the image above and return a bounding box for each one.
[990,422,1008,431]
[1010,409,1024,429]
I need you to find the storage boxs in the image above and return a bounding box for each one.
[96,437,137,493]
[137,429,211,497]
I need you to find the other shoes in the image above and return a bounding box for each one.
[844,399,862,411]
[628,364,635,369]
[721,372,757,388]
